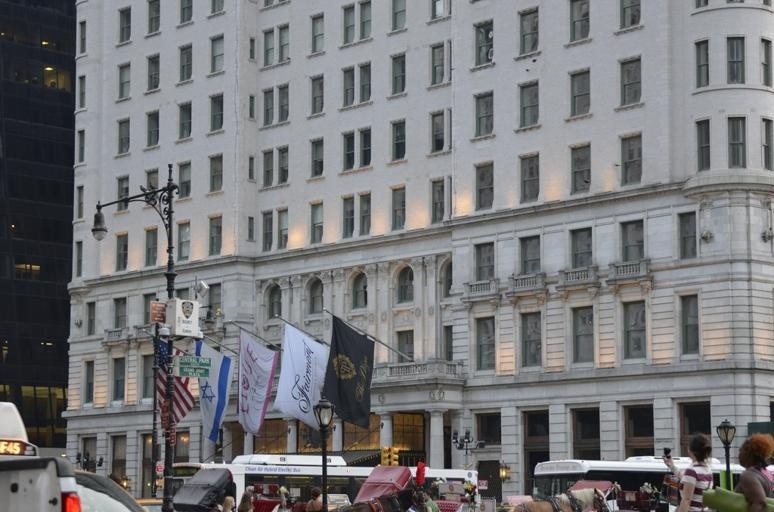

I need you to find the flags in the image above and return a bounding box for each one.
[153,338,196,429]
[238,328,280,437]
[194,338,234,443]
[272,322,331,432]
[321,316,375,430]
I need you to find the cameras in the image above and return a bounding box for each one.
[663,448,671,458]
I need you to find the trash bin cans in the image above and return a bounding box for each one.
[477,460,501,503]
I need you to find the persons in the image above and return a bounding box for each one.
[735,433,774,512]
[222,485,254,512]
[290,487,323,511]
[423,489,439,512]
[664,434,714,512]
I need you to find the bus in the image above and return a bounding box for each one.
[0,401,148,512]
[532,455,774,512]
[170,454,480,512]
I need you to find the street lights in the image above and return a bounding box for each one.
[74,451,103,472]
[716,419,736,489]
[312,394,335,512]
[92,164,179,512]
[453,429,485,454]
